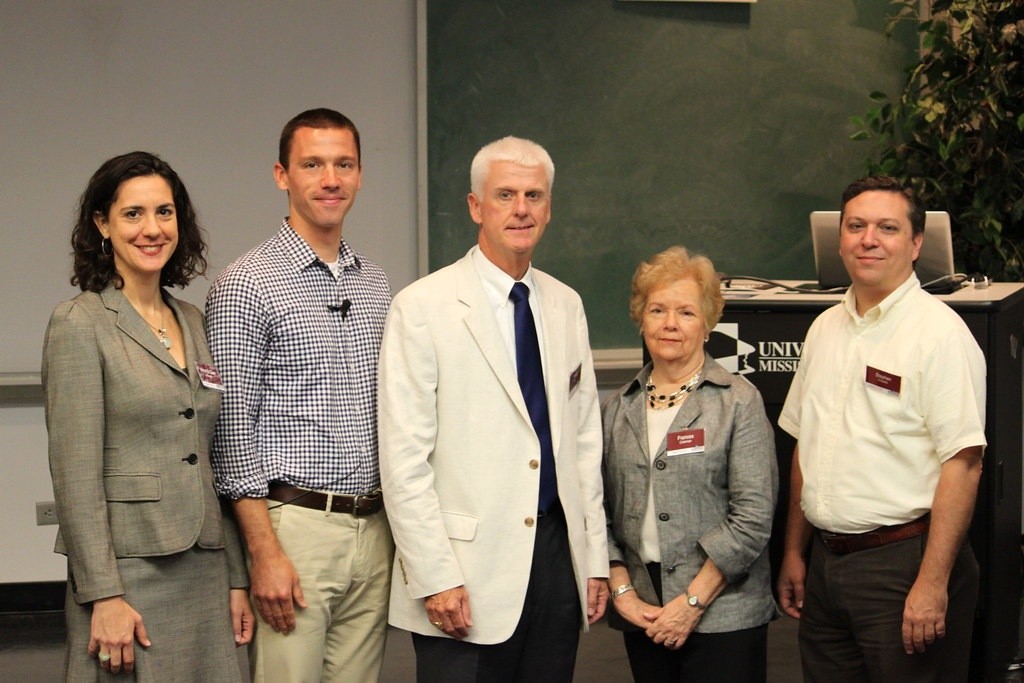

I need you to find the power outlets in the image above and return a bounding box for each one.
[35,501,58,526]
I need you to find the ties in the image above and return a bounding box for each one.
[508,283,556,514]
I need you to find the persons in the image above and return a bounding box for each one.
[377,135,610,683]
[41,151,255,683]
[777,175,987,683]
[204,109,393,682]
[601,246,779,683]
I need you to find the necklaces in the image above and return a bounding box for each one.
[145,293,171,349]
[646,370,702,411]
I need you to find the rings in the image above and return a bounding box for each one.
[432,622,443,629]
[98,653,110,661]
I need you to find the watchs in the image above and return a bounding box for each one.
[685,588,708,610]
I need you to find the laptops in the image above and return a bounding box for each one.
[811,210,967,290]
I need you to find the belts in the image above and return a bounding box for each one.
[816,519,930,556]
[268,482,382,518]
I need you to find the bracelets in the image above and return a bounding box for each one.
[611,583,635,600]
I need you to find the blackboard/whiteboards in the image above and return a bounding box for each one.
[0,0,933,387]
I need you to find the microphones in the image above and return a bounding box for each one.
[341,299,352,320]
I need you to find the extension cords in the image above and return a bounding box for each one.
[972,275,989,289]
